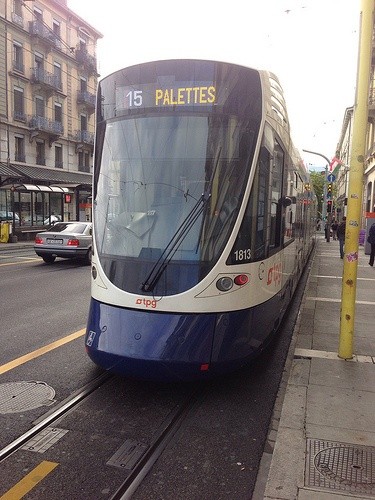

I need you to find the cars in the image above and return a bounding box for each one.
[34,221,94,263]
[24,214,62,225]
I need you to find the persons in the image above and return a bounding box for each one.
[367,223,375,268]
[324,219,338,243]
[336,216,346,260]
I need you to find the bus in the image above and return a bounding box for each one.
[86,59,317,380]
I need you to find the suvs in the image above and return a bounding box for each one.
[0,210,20,223]
